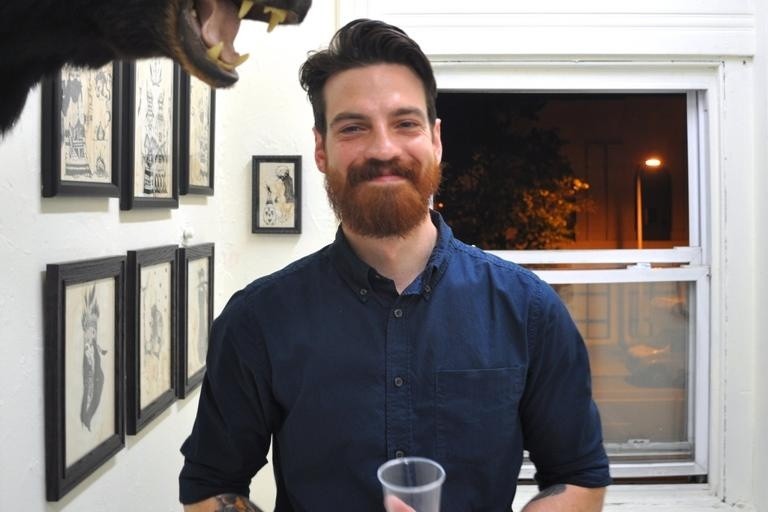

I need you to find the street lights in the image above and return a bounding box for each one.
[635,157,661,249]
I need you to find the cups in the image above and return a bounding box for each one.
[378,457,446,512]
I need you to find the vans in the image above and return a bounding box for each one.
[625,327,688,386]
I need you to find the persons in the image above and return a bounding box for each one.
[180,17,615,511]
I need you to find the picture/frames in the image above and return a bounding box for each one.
[252,154,303,233]
[180,241,214,399]
[127,242,180,437]
[43,255,127,504]
[42,56,217,211]
